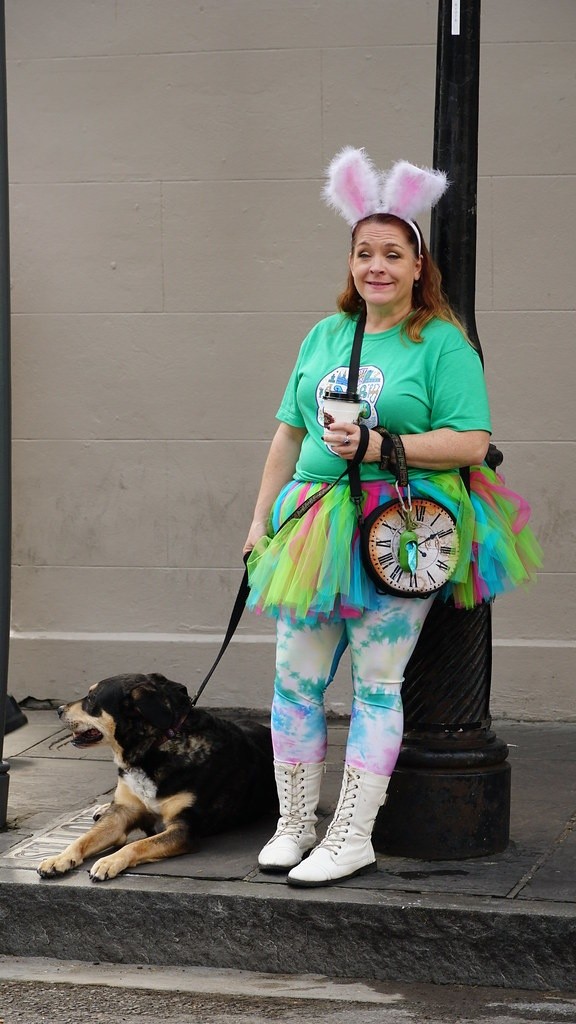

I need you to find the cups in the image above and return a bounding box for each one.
[322,391,361,447]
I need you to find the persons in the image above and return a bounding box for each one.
[242,148,546,884]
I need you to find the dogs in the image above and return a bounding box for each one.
[37,673,283,882]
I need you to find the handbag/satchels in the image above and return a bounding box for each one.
[359,496,462,599]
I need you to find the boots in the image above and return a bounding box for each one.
[258,759,328,872]
[288,763,391,888]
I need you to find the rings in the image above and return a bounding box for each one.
[345,434,350,445]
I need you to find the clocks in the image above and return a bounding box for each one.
[359,496,461,600]
